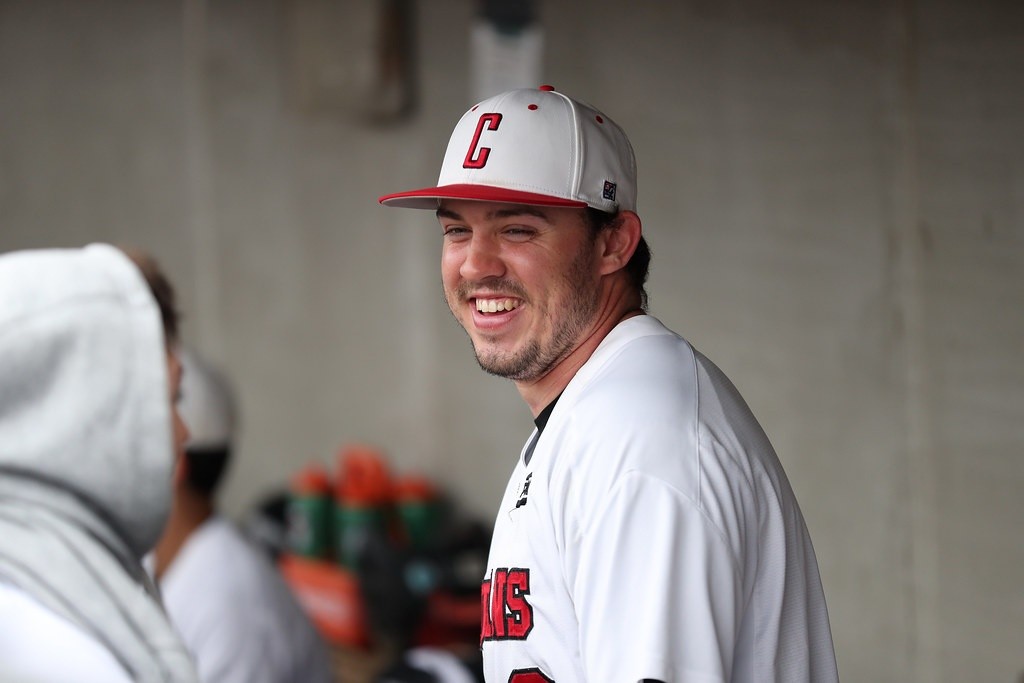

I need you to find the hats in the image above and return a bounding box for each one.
[376,84,637,216]
[177,347,235,448]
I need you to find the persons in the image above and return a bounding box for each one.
[379,84,844,683]
[140,348,333,682]
[0,241,198,683]
